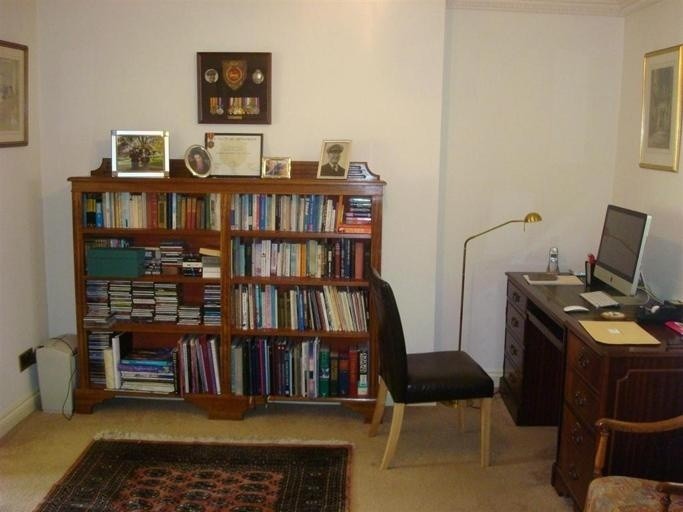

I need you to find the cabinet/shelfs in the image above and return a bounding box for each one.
[63,154,387,432]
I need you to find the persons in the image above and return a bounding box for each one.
[189,151,209,174]
[320,144,345,176]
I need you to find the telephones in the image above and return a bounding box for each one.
[546,247,560,276]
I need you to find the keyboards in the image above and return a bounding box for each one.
[579,289,620,310]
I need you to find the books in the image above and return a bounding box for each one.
[88,330,222,396]
[230,193,373,234]
[230,336,369,398]
[82,193,223,231]
[83,279,222,326]
[83,238,222,278]
[230,237,371,281]
[233,282,370,333]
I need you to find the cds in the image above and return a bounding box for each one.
[601,310,627,320]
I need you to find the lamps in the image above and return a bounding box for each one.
[435,211,543,409]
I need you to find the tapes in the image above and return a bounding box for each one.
[650,305,659,313]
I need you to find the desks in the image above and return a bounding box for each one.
[493,265,683,511]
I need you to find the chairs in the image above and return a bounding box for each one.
[581,411,683,511]
[358,264,496,472]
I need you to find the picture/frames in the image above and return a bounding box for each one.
[182,144,215,180]
[201,132,264,179]
[0,39,31,148]
[313,139,354,181]
[191,50,272,127]
[108,128,171,181]
[258,154,292,180]
[636,43,682,173]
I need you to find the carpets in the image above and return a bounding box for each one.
[22,425,360,512]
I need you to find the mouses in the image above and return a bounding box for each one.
[563,304,590,314]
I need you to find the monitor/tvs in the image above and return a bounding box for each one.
[591,203,653,307]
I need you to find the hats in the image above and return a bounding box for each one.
[326,144,344,153]
[207,70,216,75]
